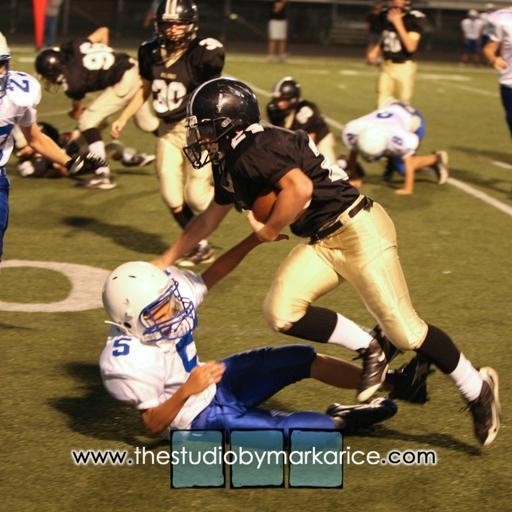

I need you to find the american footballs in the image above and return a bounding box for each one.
[253,192,312,227]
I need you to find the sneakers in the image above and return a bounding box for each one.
[120,152,156,168]
[173,242,216,267]
[83,172,117,190]
[325,397,397,435]
[467,365,503,449]
[352,325,401,403]
[431,149,448,186]
[388,354,434,404]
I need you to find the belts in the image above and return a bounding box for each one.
[308,196,368,242]
[385,58,416,62]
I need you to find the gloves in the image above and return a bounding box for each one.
[64,151,109,178]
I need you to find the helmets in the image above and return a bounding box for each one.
[151,0,199,58]
[272,76,301,110]
[101,260,198,343]
[357,123,391,161]
[34,46,67,95]
[0,32,11,61]
[182,77,261,169]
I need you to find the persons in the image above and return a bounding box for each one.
[150,76,500,446]
[99,232,433,446]
[11,0,512,267]
[0,32,110,262]
